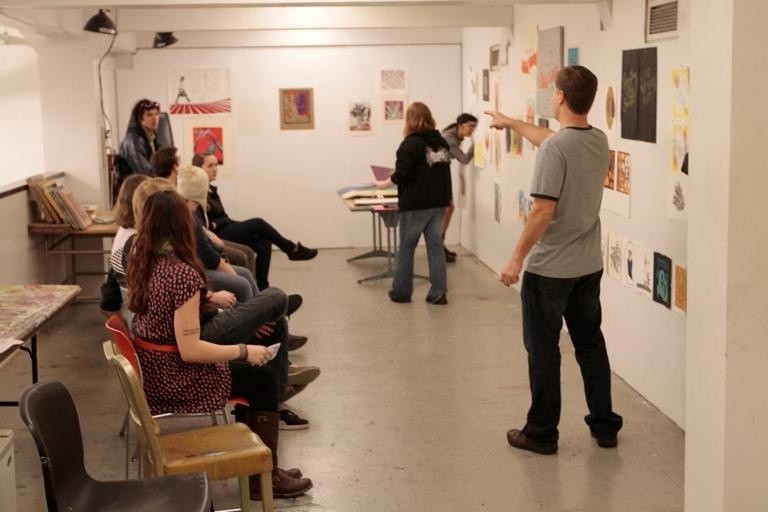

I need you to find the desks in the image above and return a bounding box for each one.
[334,179,435,288]
[0,279,86,443]
[21,207,129,311]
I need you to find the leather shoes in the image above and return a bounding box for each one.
[443,246,457,263]
[589,426,618,448]
[507,428,559,455]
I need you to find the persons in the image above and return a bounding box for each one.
[112,99,320,431]
[176,76,191,104]
[484,64,623,454]
[440,113,478,263]
[126,191,314,497]
[374,101,452,303]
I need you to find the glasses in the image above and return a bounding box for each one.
[140,100,159,110]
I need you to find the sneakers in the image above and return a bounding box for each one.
[287,364,321,402]
[286,332,308,351]
[424,294,449,304]
[287,293,303,317]
[287,241,318,261]
[386,289,411,303]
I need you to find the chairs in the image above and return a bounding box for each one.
[103,307,254,462]
[116,281,138,332]
[16,376,212,512]
[97,338,279,512]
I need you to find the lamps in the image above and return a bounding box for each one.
[81,8,120,36]
[148,30,179,51]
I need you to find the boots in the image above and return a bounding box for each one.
[230,402,313,501]
